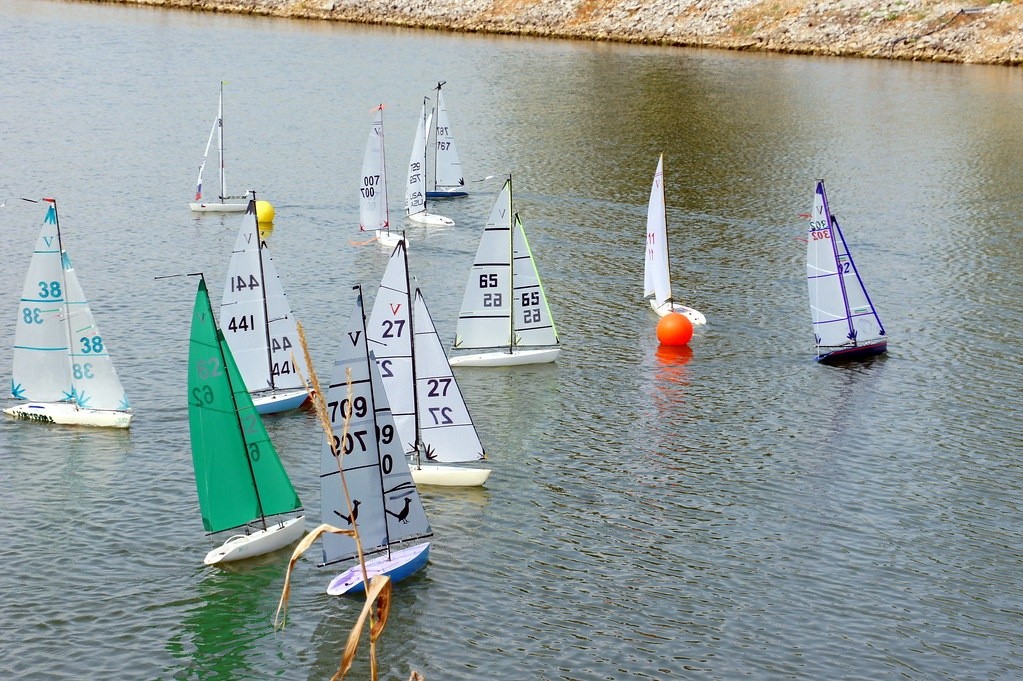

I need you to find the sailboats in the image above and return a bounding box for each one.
[185,271,308,564]
[2,195,135,432]
[448,174,564,367]
[220,189,314,415]
[319,282,437,596]
[356,230,492,489]
[356,103,411,250]
[189,79,252,212]
[643,151,707,330]
[802,177,889,365]
[424,80,471,199]
[405,94,457,227]
